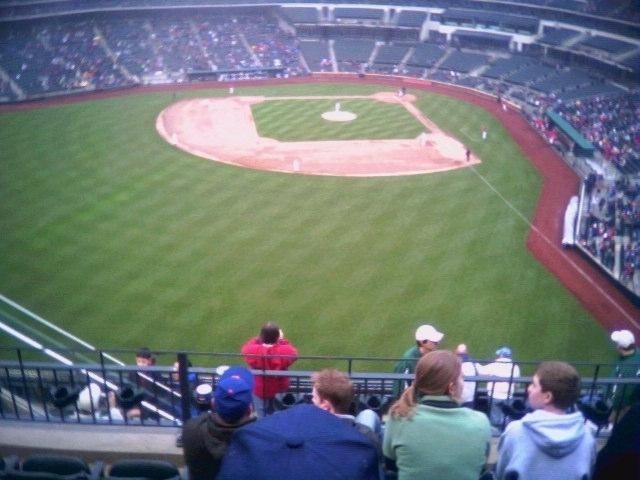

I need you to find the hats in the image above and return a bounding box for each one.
[77,383,100,410]
[214,365,253,414]
[611,330,636,348]
[457,344,467,353]
[415,325,444,342]
[495,347,512,357]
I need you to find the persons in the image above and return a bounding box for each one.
[466,151,471,162]
[497,39,640,299]
[482,129,488,139]
